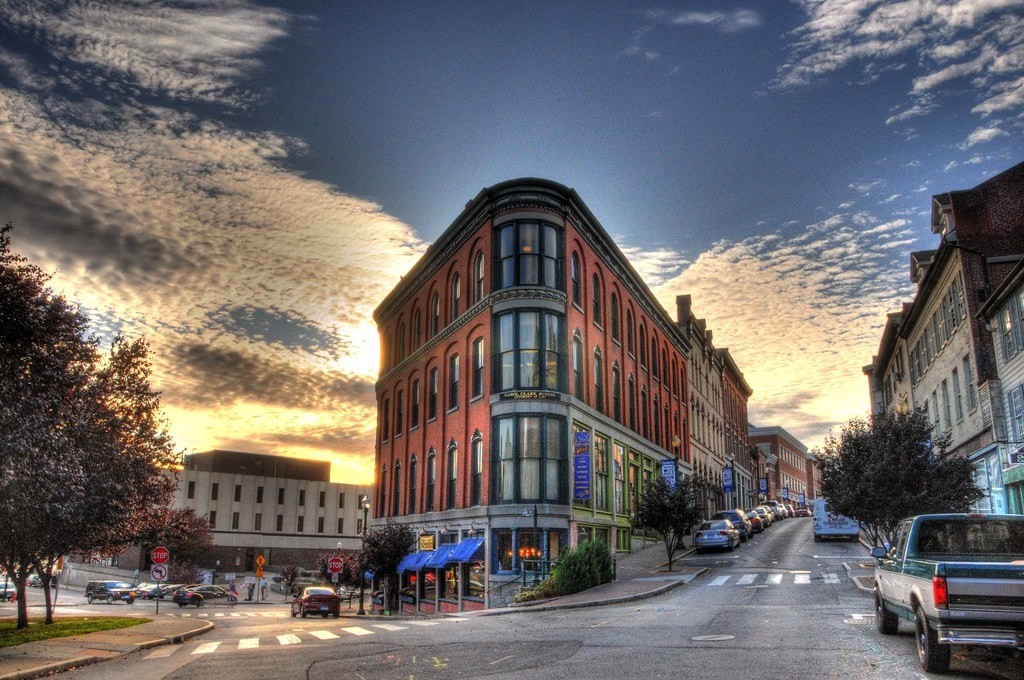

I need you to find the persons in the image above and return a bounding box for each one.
[228,577,268,602]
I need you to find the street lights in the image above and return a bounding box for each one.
[357,495,371,615]
[730,452,737,508]
[671,435,682,490]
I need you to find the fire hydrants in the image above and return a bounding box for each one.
[11,592,18,603]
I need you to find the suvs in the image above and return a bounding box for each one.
[711,509,753,543]
[84,581,137,604]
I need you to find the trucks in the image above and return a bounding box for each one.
[814,497,860,542]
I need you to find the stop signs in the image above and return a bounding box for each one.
[151,547,170,565]
[327,557,344,574]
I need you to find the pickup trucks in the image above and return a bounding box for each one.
[870,512,1024,673]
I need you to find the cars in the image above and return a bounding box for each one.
[290,586,341,618]
[28,574,44,588]
[172,585,238,608]
[744,509,765,533]
[757,500,813,528]
[694,520,740,551]
[337,587,370,602]
[134,583,185,599]
[0,582,17,598]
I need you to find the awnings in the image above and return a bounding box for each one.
[397,537,486,575]
[364,571,384,581]
